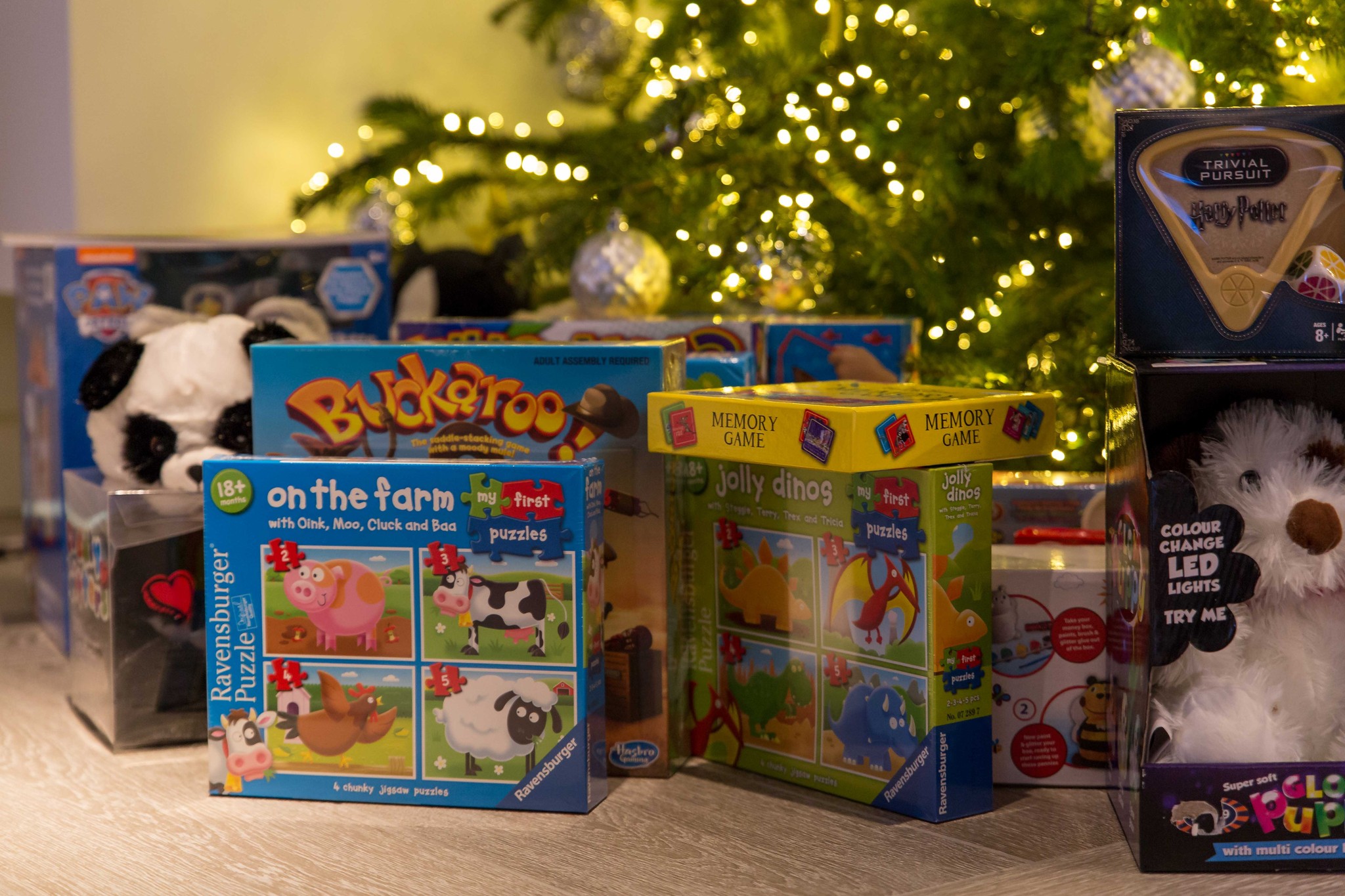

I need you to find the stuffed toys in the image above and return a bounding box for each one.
[385,233,536,343]
[77,312,302,521]
[1154,399,1343,762]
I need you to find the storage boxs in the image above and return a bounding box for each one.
[988,546,1111,787]
[55,461,211,750]
[662,451,1000,828]
[989,472,1102,543]
[200,454,610,814]
[249,338,688,779]
[1101,103,1345,876]
[645,377,1058,478]
[13,232,399,669]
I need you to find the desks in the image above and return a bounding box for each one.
[3,545,1342,896]
